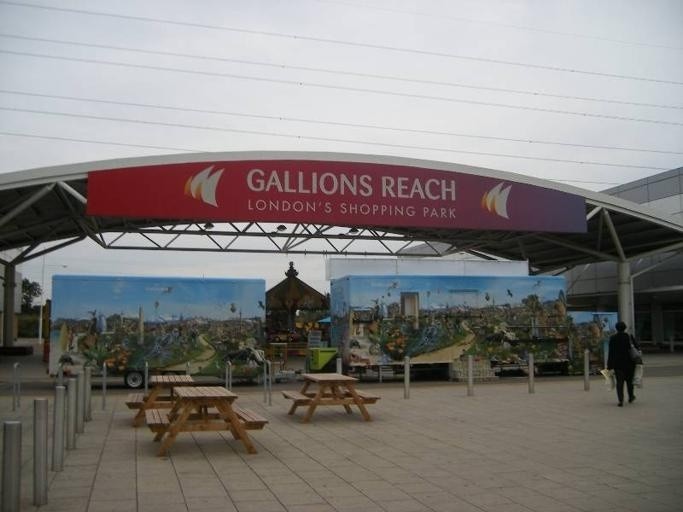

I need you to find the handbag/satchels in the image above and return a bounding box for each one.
[600,368,615,393]
[631,364,645,389]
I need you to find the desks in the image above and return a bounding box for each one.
[134,374,195,427]
[154,386,258,458]
[288,373,370,425]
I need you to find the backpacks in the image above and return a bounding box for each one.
[629,336,642,359]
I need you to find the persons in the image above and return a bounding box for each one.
[606,321,640,407]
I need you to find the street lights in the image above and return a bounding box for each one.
[38,245,68,344]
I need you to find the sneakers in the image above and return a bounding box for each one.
[617,395,636,407]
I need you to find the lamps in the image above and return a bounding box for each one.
[201,221,359,237]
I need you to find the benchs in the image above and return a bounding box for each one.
[281,390,313,405]
[125,393,146,409]
[345,389,381,404]
[232,408,268,430]
[145,408,174,432]
